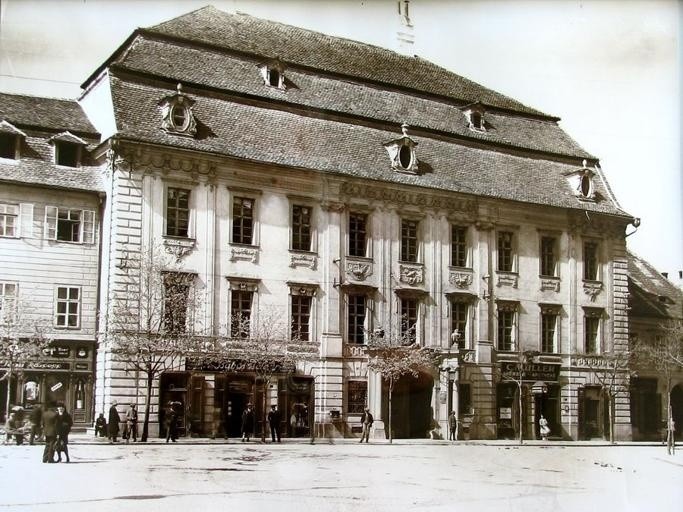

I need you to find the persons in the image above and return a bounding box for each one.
[539,413,551,440]
[5,400,284,463]
[448,411,456,440]
[359,408,373,443]
